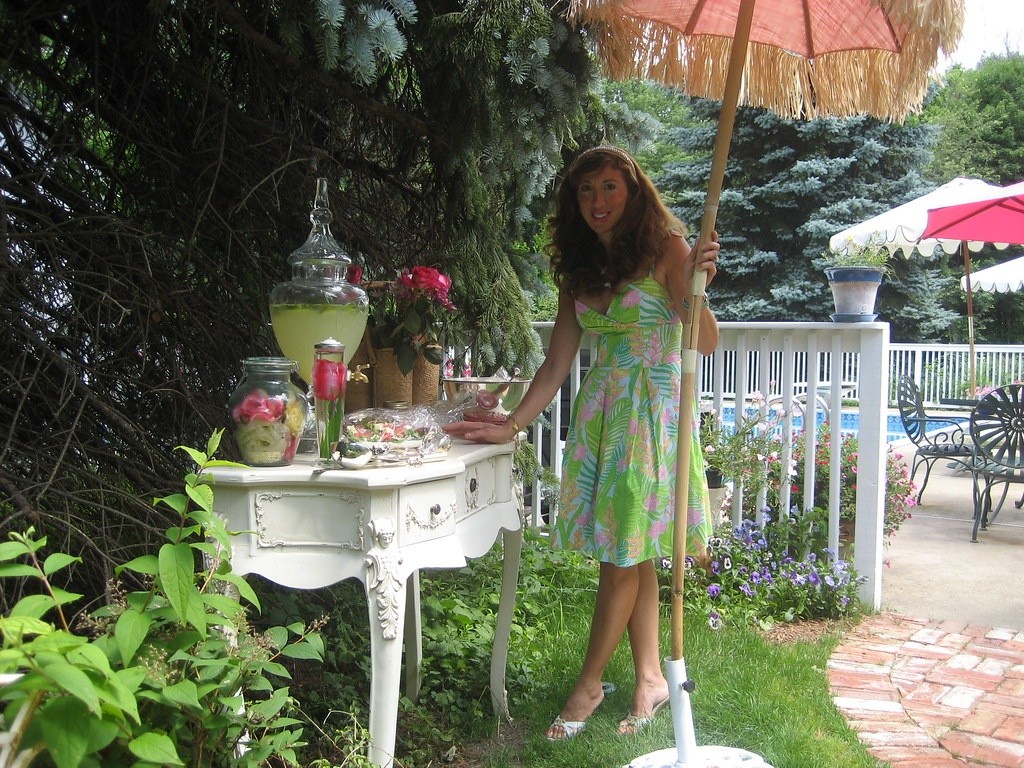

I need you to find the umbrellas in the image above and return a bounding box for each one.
[960,256,1024,293]
[568,0,964,660]
[830,176,1010,400]
[921,180,1024,245]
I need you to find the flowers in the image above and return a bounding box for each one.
[313,358,347,459]
[762,420,921,570]
[696,379,800,508]
[347,264,455,375]
[231,388,307,464]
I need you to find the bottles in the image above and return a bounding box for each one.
[269,178,370,385]
[229,356,308,467]
[313,336,345,468]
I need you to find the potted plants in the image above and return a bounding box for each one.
[821,230,901,323]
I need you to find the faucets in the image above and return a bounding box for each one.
[351,364,371,384]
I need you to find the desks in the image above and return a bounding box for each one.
[939,398,1024,526]
[199,440,523,768]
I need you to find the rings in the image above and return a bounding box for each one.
[716,256,719,262]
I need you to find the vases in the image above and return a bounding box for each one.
[228,357,309,468]
[787,516,855,561]
[314,337,346,464]
[702,469,722,489]
[709,486,728,533]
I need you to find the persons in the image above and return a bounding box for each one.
[439,146,720,742]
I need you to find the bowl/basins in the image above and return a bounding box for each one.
[443,377,532,426]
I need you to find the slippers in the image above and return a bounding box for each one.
[619,694,670,735]
[546,714,587,740]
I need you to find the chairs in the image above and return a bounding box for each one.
[897,374,995,520]
[968,383,1024,544]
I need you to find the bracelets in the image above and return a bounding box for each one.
[682,291,709,311]
[508,415,519,431]
